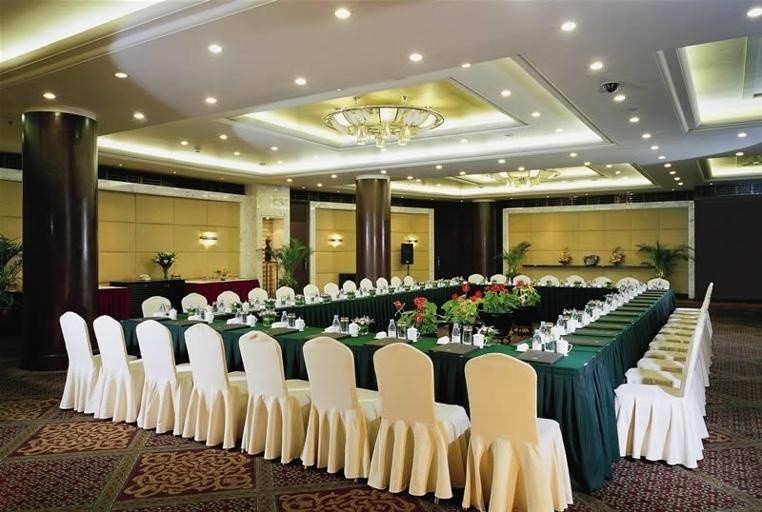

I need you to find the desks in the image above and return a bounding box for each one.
[120,282,674,494]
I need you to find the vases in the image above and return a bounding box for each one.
[514,308,539,324]
[163,268,169,279]
[485,313,512,336]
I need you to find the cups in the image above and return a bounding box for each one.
[288,313,295,327]
[213,302,218,312]
[311,297,314,303]
[295,317,305,330]
[545,323,553,336]
[408,326,420,342]
[551,325,560,339]
[473,331,487,349]
[341,317,349,334]
[545,335,555,353]
[398,322,406,339]
[241,312,247,323]
[246,313,257,326]
[462,326,472,346]
[206,314,214,324]
[281,296,286,306]
[556,337,573,356]
[349,323,360,335]
[170,308,177,319]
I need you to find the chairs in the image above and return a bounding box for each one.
[512,276,531,285]
[404,276,414,286]
[324,283,339,297]
[136,320,191,436]
[360,278,372,289]
[238,331,309,460]
[564,275,586,287]
[89,316,143,422]
[303,284,320,302]
[181,293,208,313]
[302,337,381,478]
[614,281,715,468]
[591,276,612,288]
[368,344,464,499]
[247,288,268,304]
[216,291,240,312]
[391,277,401,288]
[464,354,573,511]
[648,278,670,289]
[618,277,640,291]
[276,287,295,302]
[538,275,559,286]
[343,280,356,293]
[376,278,388,291]
[490,275,506,284]
[141,296,172,320]
[184,323,246,448]
[59,312,136,412]
[468,274,484,284]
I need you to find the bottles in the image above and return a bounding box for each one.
[332,314,342,334]
[388,319,397,340]
[571,310,577,322]
[281,311,290,328]
[539,321,547,333]
[532,330,542,352]
[194,306,202,319]
[584,305,589,318]
[452,322,461,344]
[234,308,243,322]
[556,315,564,336]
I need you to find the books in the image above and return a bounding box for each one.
[363,337,411,349]
[304,332,352,343]
[616,289,668,312]
[606,311,642,317]
[515,349,563,366]
[428,343,480,357]
[564,338,608,347]
[262,328,299,336]
[129,317,171,323]
[163,320,209,326]
[585,323,626,330]
[213,323,250,332]
[595,317,634,323]
[571,328,618,338]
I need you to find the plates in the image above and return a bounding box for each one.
[373,337,385,339]
[413,340,417,342]
[516,349,531,353]
[321,331,326,333]
[352,335,358,337]
[288,327,296,329]
[436,342,445,345]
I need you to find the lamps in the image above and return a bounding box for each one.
[320,96,446,148]
[405,235,419,247]
[488,169,561,185]
[198,232,217,248]
[329,233,343,247]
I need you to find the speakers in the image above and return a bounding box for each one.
[401,244,413,264]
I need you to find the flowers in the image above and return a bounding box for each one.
[482,283,516,312]
[393,298,437,334]
[152,250,179,267]
[441,284,482,322]
[512,281,542,307]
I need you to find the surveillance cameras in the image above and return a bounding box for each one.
[603,82,620,92]
